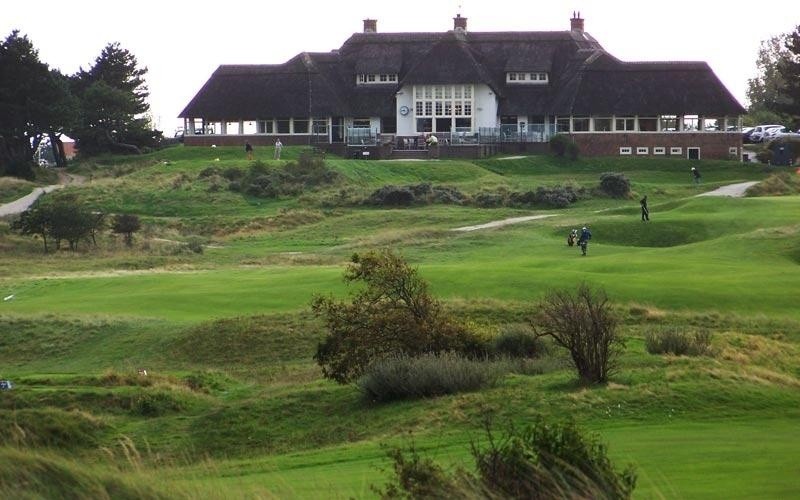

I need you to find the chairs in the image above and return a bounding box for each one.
[403,138,426,150]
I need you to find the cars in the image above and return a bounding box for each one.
[742,125,800,143]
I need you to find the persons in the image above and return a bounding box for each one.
[425,134,438,148]
[567,229,577,247]
[640,195,650,221]
[244,140,253,161]
[691,166,701,183]
[274,138,283,160]
[577,227,592,256]
